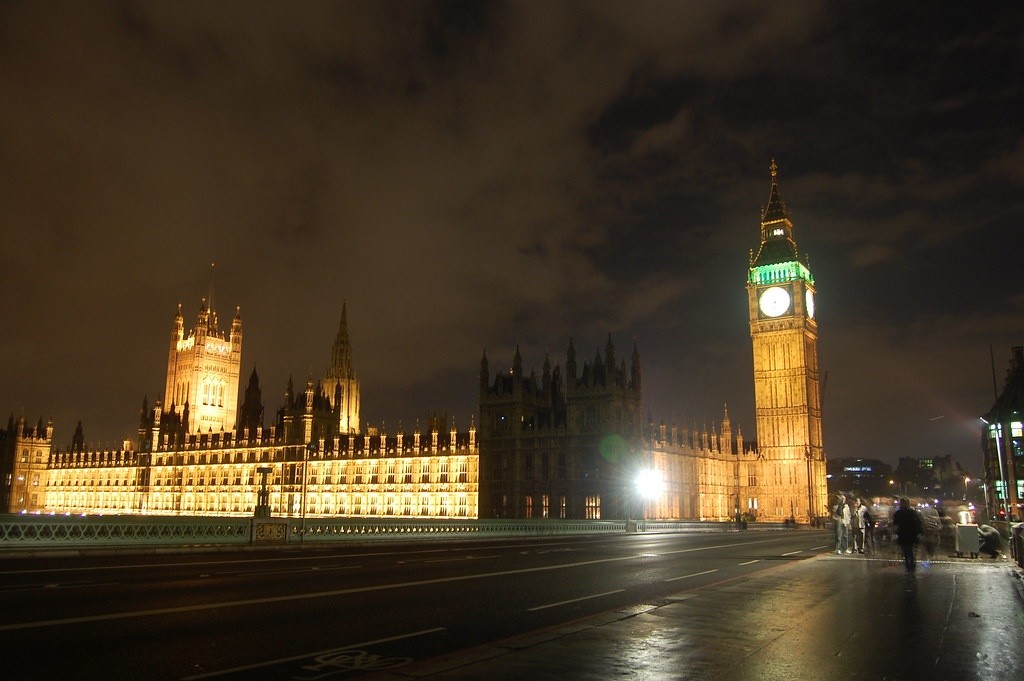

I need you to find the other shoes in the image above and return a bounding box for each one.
[836,550,841,555]
[852,551,857,553]
[843,550,851,554]
[859,551,864,554]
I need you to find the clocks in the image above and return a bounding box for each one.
[759,287,791,317]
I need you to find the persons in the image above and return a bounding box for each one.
[735,512,748,530]
[977,524,1001,559]
[785,518,795,529]
[811,495,976,555]
[892,497,926,572]
